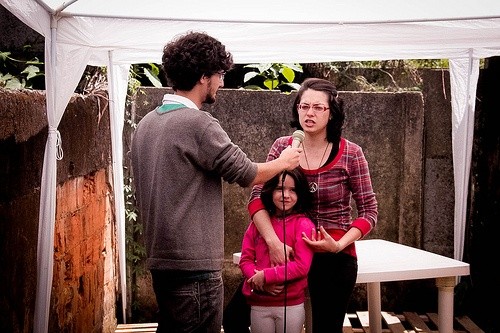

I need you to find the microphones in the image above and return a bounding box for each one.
[281,129,306,181]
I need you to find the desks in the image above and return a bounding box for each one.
[232,238,470,333]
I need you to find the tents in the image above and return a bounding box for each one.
[0,1,500,333]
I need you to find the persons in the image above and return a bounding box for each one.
[131,32,301,333]
[240,168,316,333]
[248,77,377,333]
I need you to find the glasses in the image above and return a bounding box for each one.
[296,104,330,113]
[215,71,226,79]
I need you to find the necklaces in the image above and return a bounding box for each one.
[301,140,330,192]
